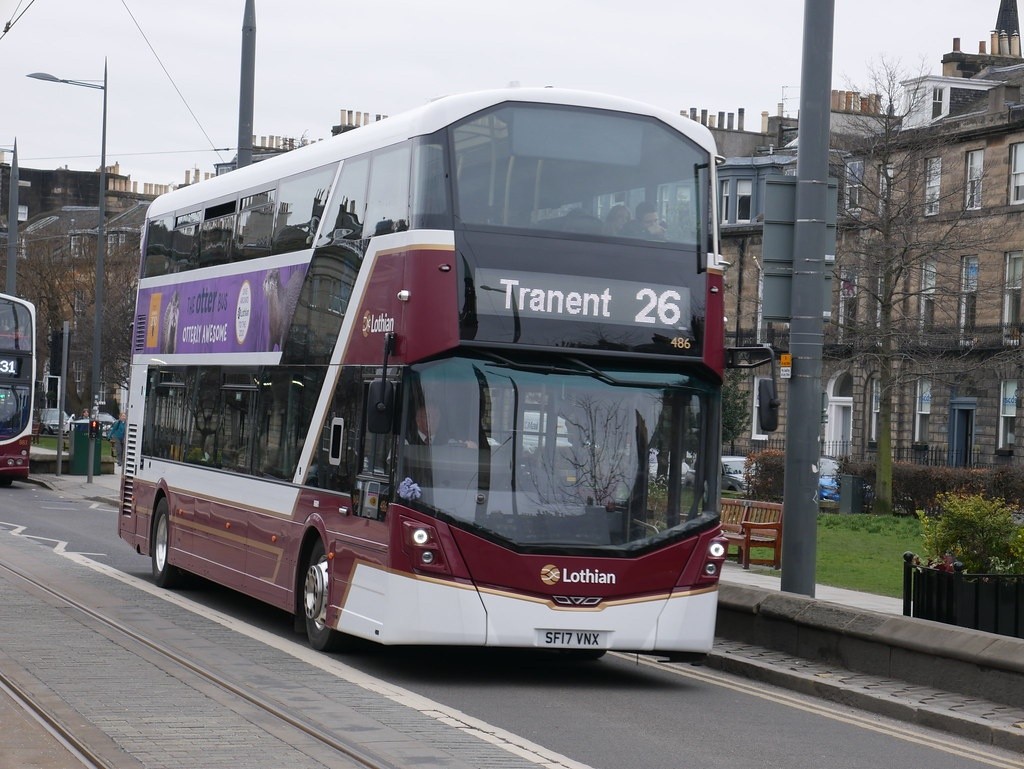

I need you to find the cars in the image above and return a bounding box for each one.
[686,454,764,492]
[648,449,692,485]
[819,457,878,501]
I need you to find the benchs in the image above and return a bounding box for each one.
[680,497,784,569]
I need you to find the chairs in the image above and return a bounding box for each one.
[468,189,667,239]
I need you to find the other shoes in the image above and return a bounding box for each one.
[117,462,121,466]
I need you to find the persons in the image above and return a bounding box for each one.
[76,408,90,432]
[386,404,479,463]
[106,413,127,466]
[622,200,669,240]
[604,203,631,230]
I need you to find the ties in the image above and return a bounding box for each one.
[424,437,431,444]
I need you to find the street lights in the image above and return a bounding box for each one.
[27,56,108,484]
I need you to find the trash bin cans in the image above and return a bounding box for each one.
[69,420,103,476]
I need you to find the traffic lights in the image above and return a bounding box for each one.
[90,421,98,436]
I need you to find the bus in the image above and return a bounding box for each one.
[118,85,778,654]
[0,293,36,487]
